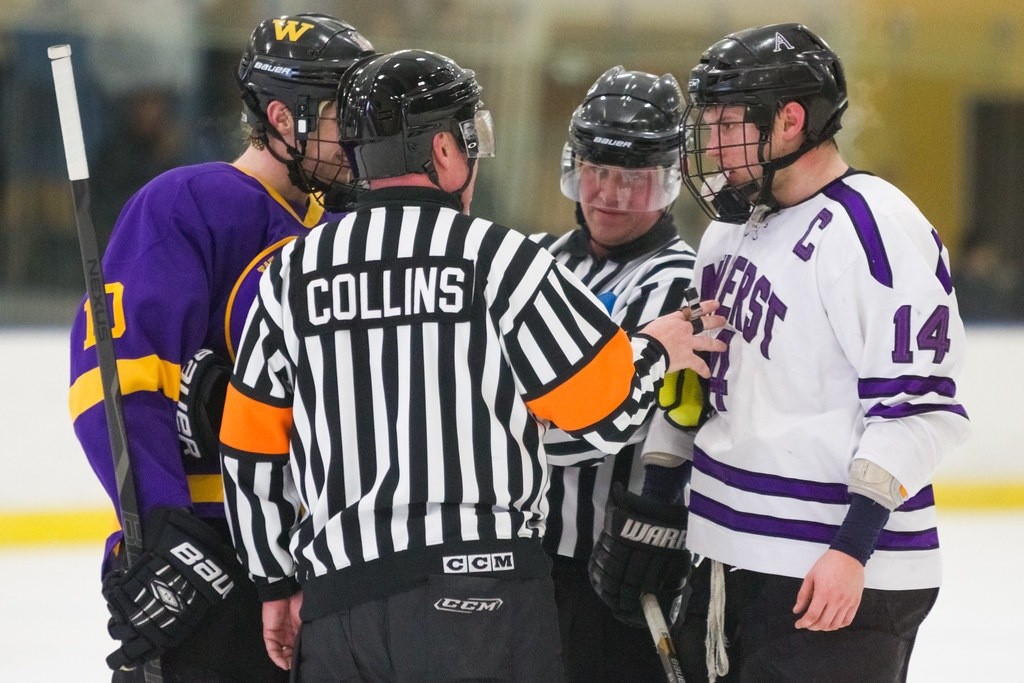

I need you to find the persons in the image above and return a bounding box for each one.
[220,50,726,683]
[71,14,379,683]
[588,23,976,683]
[519,63,696,683]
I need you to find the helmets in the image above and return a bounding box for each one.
[236,13,375,137]
[560,65,694,212]
[678,22,848,135]
[336,48,496,180]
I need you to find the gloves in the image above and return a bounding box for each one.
[587,481,691,629]
[102,507,248,672]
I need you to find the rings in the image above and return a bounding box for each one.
[688,318,703,334]
[689,303,703,319]
[678,306,692,319]
[281,645,291,650]
